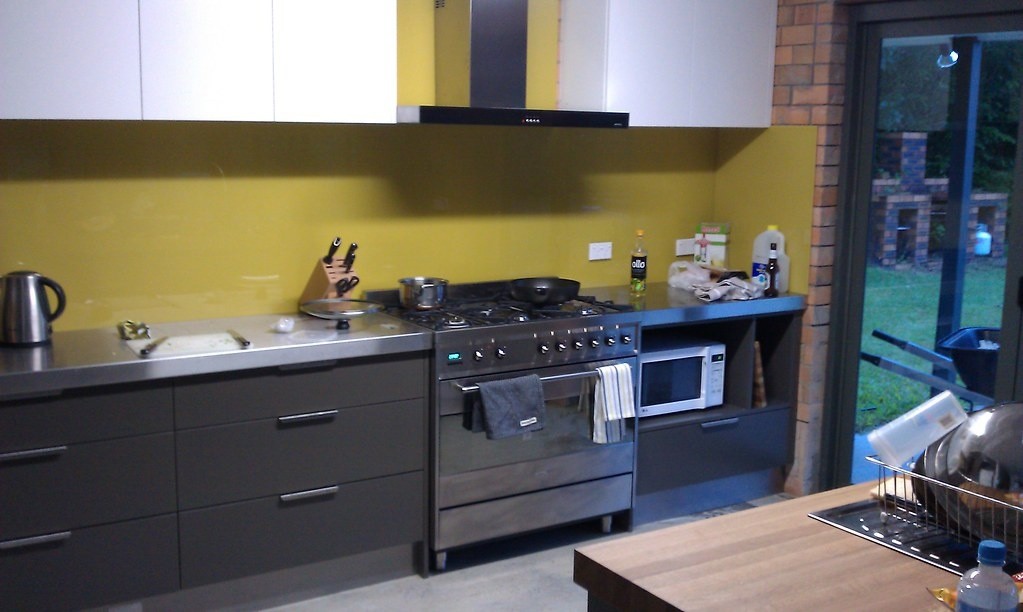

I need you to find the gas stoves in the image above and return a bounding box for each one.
[380,293,640,334]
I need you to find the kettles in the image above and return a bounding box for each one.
[0,270,66,347]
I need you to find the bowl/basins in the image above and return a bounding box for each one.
[911,403,1023,565]
[509,277,580,304]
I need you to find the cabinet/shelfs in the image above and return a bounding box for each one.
[630,310,804,529]
[1,1,272,122]
[554,0,779,127]
[0,355,432,612]
[273,1,401,122]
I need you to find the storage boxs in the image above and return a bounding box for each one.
[694,221,730,268]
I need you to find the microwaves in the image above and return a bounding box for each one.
[640,340,727,417]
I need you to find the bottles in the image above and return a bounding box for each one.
[765,243,780,297]
[956,540,1020,612]
[751,225,790,293]
[630,230,647,295]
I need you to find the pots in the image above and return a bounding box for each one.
[397,276,449,309]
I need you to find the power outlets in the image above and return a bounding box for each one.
[677,239,695,255]
[588,242,613,261]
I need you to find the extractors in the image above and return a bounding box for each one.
[397,0,630,129]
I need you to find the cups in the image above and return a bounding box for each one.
[868,390,968,469]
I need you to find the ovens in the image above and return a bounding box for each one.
[428,312,641,572]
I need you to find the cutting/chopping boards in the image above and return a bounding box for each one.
[125,332,254,358]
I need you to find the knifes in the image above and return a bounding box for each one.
[324,237,357,274]
[227,329,250,347]
[141,336,169,354]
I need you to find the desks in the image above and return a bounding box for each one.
[574,475,1023,612]
